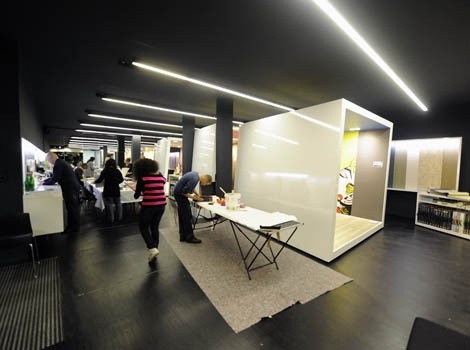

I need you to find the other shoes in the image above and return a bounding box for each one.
[186,236,201,243]
[143,248,159,256]
[147,248,159,263]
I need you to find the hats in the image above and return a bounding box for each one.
[132,155,138,160]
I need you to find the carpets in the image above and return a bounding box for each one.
[0,257,64,350]
[158,220,354,335]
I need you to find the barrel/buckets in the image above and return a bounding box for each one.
[224,193,242,211]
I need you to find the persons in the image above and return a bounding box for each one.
[174,172,213,244]
[92,159,125,223]
[42,152,84,235]
[68,156,96,210]
[132,158,167,262]
[125,158,132,177]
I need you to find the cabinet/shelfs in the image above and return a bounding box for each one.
[415,191,470,241]
[168,173,182,200]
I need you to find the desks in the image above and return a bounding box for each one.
[84,177,144,225]
[25,183,68,240]
[0,213,40,278]
[189,195,301,280]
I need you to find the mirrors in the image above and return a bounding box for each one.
[386,136,463,193]
